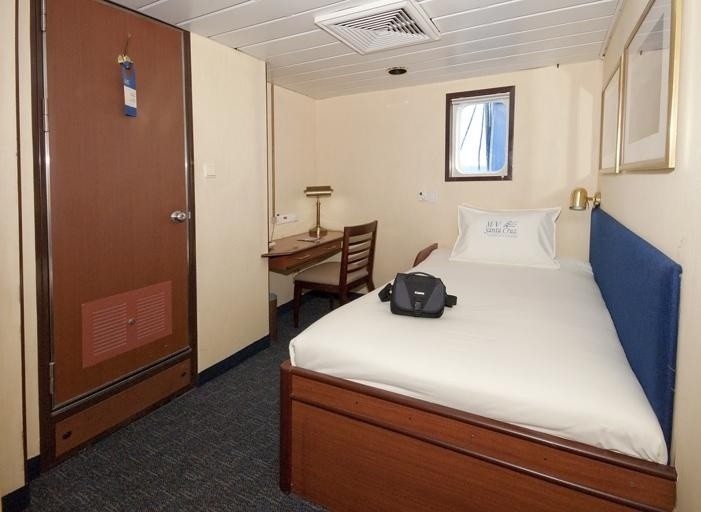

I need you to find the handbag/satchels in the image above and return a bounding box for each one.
[378,272,456,318]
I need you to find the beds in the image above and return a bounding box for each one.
[279,247,676,512]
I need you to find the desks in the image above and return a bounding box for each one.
[268,227,346,276]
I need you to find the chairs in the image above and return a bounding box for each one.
[292,219,380,328]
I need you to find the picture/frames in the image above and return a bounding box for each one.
[444,85,514,183]
[598,56,622,176]
[621,0,678,172]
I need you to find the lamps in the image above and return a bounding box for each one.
[568,187,600,212]
[304,185,335,235]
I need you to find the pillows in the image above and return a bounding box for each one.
[450,202,560,268]
